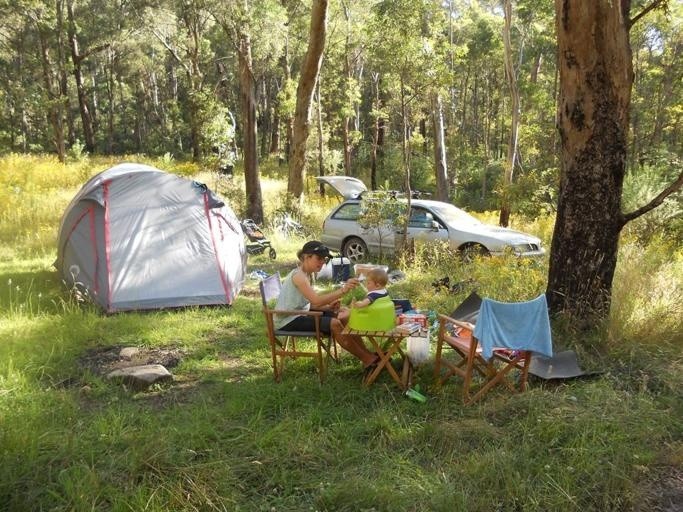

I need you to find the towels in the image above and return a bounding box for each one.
[472,294,553,363]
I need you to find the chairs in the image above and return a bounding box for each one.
[433,297,532,408]
[259,271,337,384]
[348,295,395,331]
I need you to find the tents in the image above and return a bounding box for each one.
[53,161,249,313]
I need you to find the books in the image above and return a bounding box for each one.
[395,312,427,335]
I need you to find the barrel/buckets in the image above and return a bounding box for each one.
[407,332,430,363]
[332,252,351,281]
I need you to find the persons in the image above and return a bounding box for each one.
[271,241,393,371]
[351,269,389,308]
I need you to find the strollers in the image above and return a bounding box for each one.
[240,218,277,259]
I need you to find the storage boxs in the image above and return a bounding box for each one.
[331,257,351,281]
[355,264,388,278]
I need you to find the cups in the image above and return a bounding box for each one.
[402,385,428,405]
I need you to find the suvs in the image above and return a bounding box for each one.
[315,174,545,266]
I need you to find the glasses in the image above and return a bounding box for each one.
[305,245,330,253]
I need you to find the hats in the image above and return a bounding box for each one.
[302,240,333,259]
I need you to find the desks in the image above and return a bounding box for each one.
[340,316,428,391]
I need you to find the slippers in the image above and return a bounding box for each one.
[364,352,392,372]
